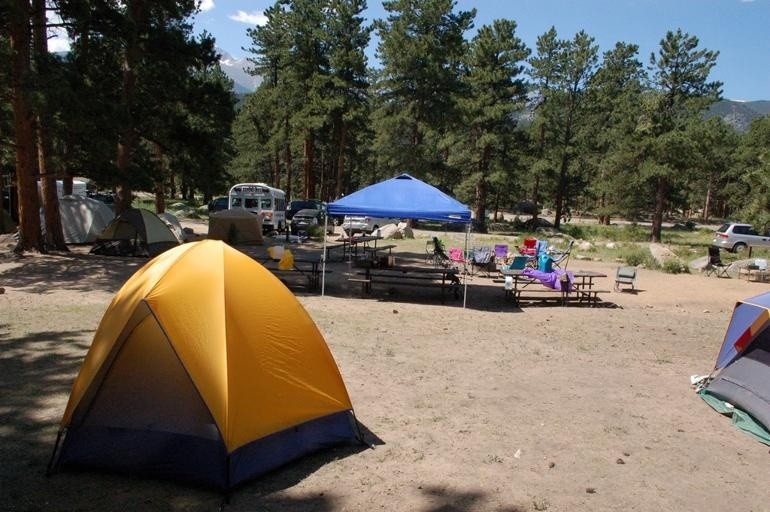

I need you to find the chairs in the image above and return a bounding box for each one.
[614,266,637,294]
[739,259,767,281]
[707,246,732,279]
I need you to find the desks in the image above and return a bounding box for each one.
[335,235,384,262]
[256,255,323,293]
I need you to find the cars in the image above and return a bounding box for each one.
[208,197,228,211]
[712,223,770,253]
[286,200,401,237]
[507,199,543,215]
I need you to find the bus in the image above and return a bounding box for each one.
[229,182,286,235]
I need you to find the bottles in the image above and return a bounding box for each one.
[0,287,9,335]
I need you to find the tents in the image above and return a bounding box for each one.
[83,207,185,260]
[204,204,265,246]
[157,212,193,241]
[688,291,770,447]
[45,234,366,503]
[12,192,120,246]
